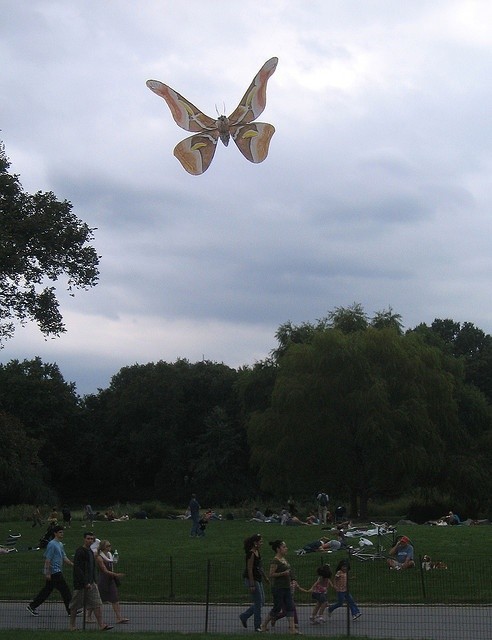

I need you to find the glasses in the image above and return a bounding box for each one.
[59,532,62,533]
[256,533,261,539]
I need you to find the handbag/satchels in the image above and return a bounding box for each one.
[114,575,121,588]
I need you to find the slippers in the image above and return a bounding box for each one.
[116,617,130,624]
[85,619,96,623]
[104,625,114,630]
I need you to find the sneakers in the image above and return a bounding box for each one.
[326,607,332,617]
[239,615,247,628]
[271,616,276,627]
[68,610,84,617]
[289,630,302,635]
[352,612,362,620]
[25,605,41,616]
[288,623,300,628]
[389,566,393,570]
[317,615,327,623]
[308,615,317,625]
[255,626,262,632]
[260,625,267,634]
[395,565,401,570]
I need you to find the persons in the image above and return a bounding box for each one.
[105,507,114,521]
[96,539,129,624]
[119,511,128,521]
[295,536,325,554]
[80,502,94,528]
[49,508,59,526]
[307,564,334,626]
[316,489,329,528]
[26,526,84,620]
[94,508,101,522]
[265,495,320,526]
[31,504,43,528]
[259,539,305,636]
[320,538,342,554]
[246,507,264,523]
[448,511,459,525]
[184,494,200,540]
[61,504,72,530]
[330,520,392,530]
[69,531,114,631]
[238,532,268,634]
[198,514,210,537]
[387,537,415,570]
[325,561,362,622]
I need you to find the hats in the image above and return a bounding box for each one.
[400,535,410,544]
